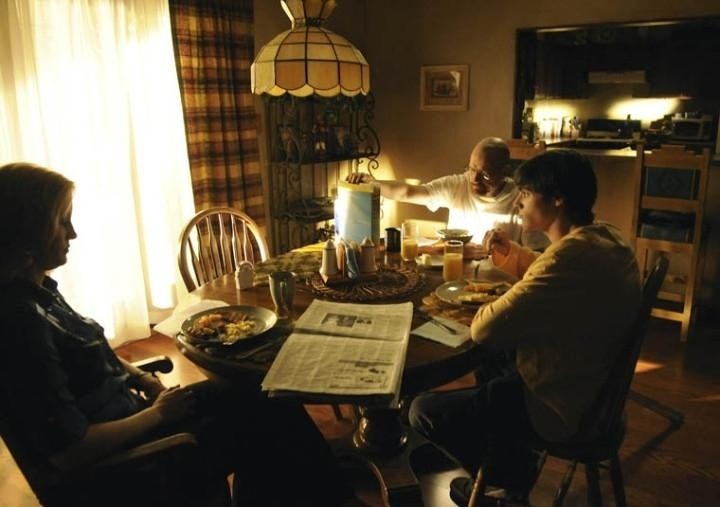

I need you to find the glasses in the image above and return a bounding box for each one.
[468,169,495,182]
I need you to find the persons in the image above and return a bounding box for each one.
[409,147,643,505]
[346,136,523,387]
[0,162,350,507]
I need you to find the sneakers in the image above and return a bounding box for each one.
[450,477,528,507]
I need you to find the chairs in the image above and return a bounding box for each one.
[179,206,345,422]
[467,250,668,506]
[90,355,197,473]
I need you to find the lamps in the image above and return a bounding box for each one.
[250,0,370,98]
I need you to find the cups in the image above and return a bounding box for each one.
[442,240,464,282]
[384,227,400,253]
[401,223,420,262]
[267,271,293,321]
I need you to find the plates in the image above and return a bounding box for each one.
[434,276,514,310]
[182,304,279,345]
[414,253,445,270]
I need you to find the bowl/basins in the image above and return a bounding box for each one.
[439,229,474,247]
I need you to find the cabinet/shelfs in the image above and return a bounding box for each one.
[631,142,712,323]
[262,91,381,258]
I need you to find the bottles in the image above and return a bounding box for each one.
[236,260,254,292]
[520,106,538,147]
[321,237,379,275]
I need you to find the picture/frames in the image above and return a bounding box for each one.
[420,65,470,112]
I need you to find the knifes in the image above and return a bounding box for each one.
[411,307,460,336]
[233,335,289,360]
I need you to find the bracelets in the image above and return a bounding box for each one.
[133,371,148,391]
